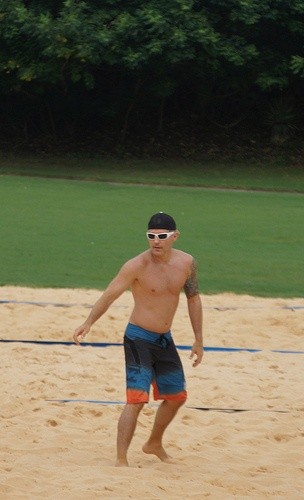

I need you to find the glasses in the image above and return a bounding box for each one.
[146,231,174,240]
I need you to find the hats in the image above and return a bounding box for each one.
[148,212,176,230]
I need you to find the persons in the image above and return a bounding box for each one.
[74,213,204,466]
